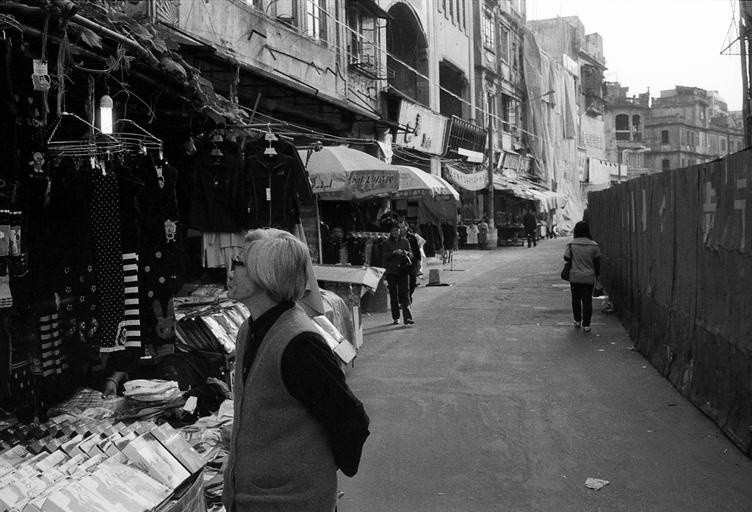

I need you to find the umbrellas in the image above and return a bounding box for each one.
[299,146,399,265]
[393,164,445,199]
[426,173,460,200]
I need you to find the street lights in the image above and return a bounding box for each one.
[485,89,497,249]
[617,144,645,183]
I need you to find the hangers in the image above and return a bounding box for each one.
[47,73,163,157]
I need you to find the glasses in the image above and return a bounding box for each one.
[231,256,244,271]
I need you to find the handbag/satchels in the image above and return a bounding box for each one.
[561,263,572,280]
[384,255,412,278]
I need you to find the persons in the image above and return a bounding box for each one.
[400,220,421,308]
[564,221,602,332]
[523,209,537,247]
[382,223,415,325]
[221,228,369,512]
